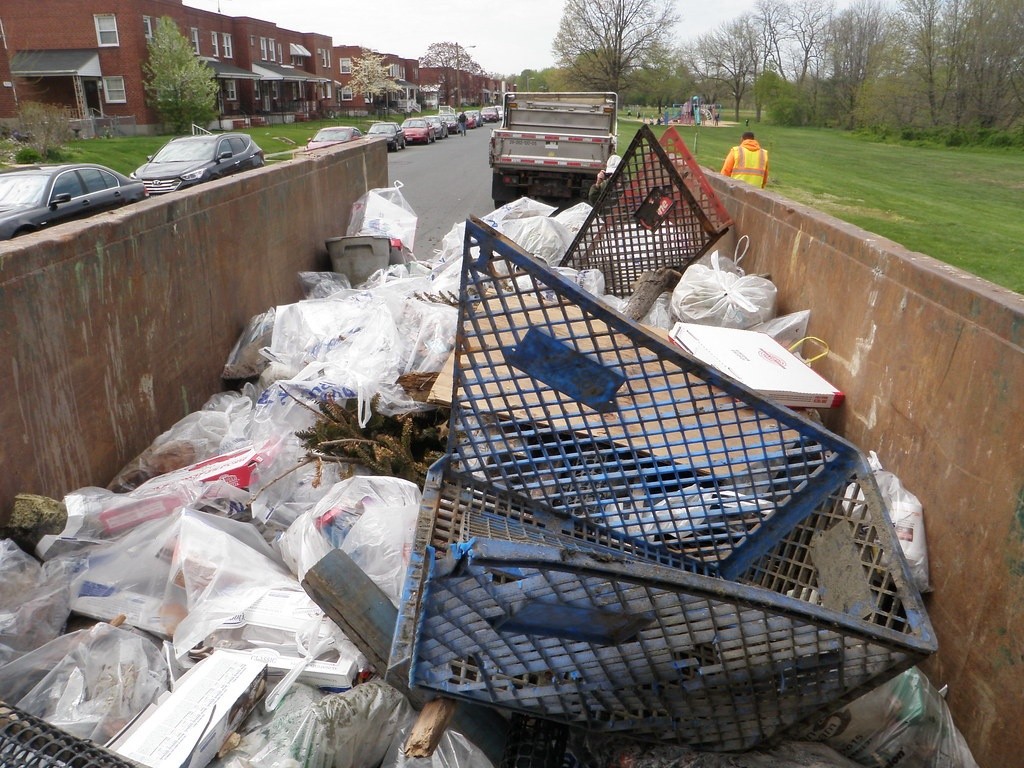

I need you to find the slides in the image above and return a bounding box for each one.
[656,110,681,123]
[693,105,701,126]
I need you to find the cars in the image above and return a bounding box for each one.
[440,115,459,134]
[400,118,435,145]
[364,122,405,152]
[306,126,363,150]
[421,116,448,140]
[0,163,149,241]
[458,105,502,129]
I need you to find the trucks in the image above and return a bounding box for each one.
[488,91,618,211]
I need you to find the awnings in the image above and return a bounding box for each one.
[252,63,308,82]
[290,44,304,55]
[10,51,102,77]
[296,45,311,57]
[200,61,264,80]
[288,68,327,82]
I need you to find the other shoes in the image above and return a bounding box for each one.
[461,134,466,136]
[715,125,718,126]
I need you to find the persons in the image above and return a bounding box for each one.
[589,155,630,221]
[746,118,749,126]
[720,132,768,189]
[636,105,641,119]
[715,112,719,126]
[458,111,468,137]
[627,108,632,118]
[650,118,654,125]
[660,116,664,121]
[656,119,660,125]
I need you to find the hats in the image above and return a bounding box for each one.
[702,112,704,114]
[605,155,622,173]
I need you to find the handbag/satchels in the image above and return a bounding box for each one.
[667,322,846,411]
[346,179,418,253]
[669,233,777,331]
[457,123,463,133]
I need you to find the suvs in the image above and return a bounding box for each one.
[128,133,265,198]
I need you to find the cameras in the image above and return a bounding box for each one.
[604,173,612,180]
